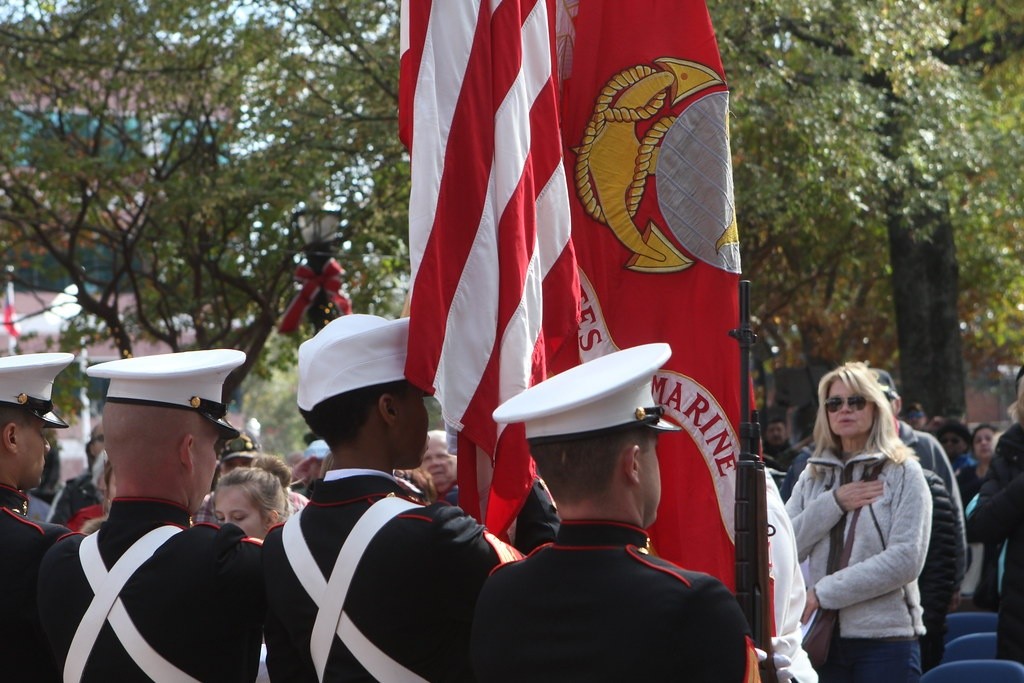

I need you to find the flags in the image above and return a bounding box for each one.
[398,0,819,683]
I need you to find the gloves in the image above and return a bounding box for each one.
[753,637,794,683]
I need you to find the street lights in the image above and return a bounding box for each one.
[292,185,344,334]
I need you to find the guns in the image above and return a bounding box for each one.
[729,276,777,682]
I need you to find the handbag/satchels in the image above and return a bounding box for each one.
[801,605,838,668]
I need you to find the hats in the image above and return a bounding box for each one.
[296,313,417,412]
[868,367,900,400]
[218,429,260,462]
[85,348,246,441]
[0,353,75,428]
[304,439,332,459]
[492,344,683,442]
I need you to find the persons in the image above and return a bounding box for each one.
[763,360,1024,683]
[0,353,115,683]
[469,343,794,683]
[261,314,561,683]
[36,349,333,683]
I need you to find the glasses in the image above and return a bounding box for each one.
[883,392,894,402]
[908,411,924,419]
[823,394,872,412]
[90,433,104,444]
